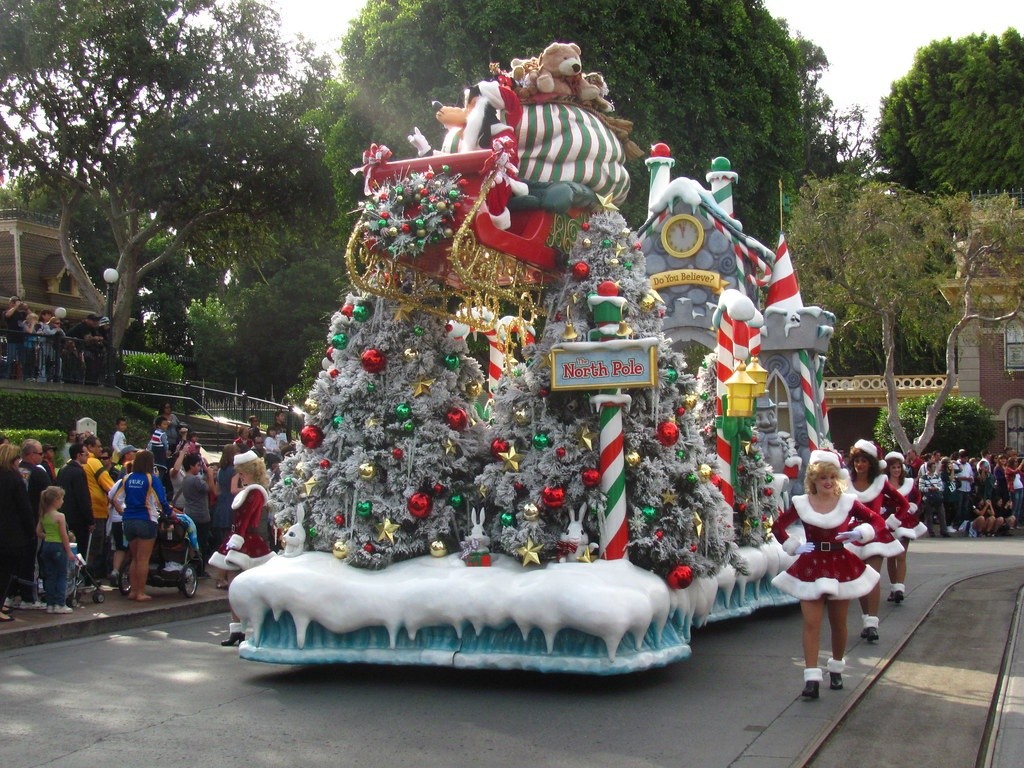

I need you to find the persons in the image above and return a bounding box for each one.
[36,486,75,613]
[153,401,180,451]
[0,411,296,621]
[207,451,279,646]
[771,449,883,699]
[904,446,1024,538]
[884,451,922,605]
[0,296,110,385]
[843,440,911,642]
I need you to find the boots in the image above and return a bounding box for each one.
[861,615,868,638]
[887,582,895,601]
[802,668,823,698]
[221,623,245,646]
[894,583,904,604]
[864,616,879,641]
[826,658,846,689]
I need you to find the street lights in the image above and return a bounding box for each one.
[53,308,67,382]
[102,268,119,388]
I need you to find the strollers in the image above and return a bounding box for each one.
[65,520,105,607]
[119,510,204,599]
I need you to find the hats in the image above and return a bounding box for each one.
[854,438,887,468]
[478,80,522,135]
[809,449,849,480]
[233,450,258,465]
[42,444,57,452]
[884,451,907,472]
[121,445,139,456]
[88,314,99,320]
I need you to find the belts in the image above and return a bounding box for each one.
[816,542,843,551]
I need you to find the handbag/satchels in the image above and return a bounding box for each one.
[926,491,943,507]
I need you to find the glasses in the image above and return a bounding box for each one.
[32,452,43,456]
[98,457,110,460]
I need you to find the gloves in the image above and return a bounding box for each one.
[226,542,237,550]
[835,531,861,544]
[407,127,431,156]
[794,542,815,553]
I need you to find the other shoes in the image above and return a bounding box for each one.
[946,525,956,533]
[940,532,950,537]
[197,572,212,579]
[0,597,73,622]
[216,579,228,588]
[127,592,136,600]
[929,532,936,537]
[137,593,151,601]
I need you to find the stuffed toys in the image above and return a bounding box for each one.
[408,81,521,230]
[531,42,599,101]
